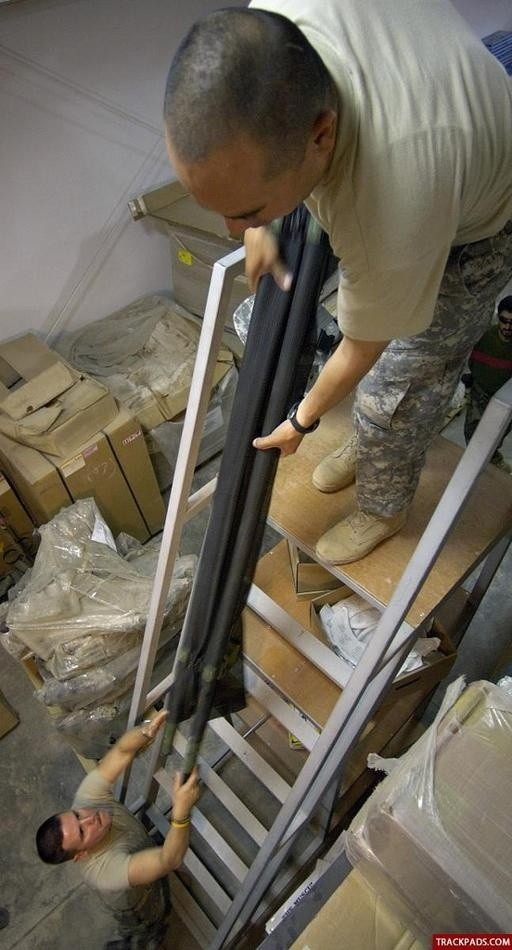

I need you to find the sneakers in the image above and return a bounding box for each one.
[311,434,356,493]
[314,509,408,566]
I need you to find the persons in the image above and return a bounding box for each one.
[35,690,201,950]
[160,1,511,570]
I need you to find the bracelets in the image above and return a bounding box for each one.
[169,815,192,829]
[285,401,321,434]
[140,718,153,742]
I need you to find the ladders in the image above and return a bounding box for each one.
[117,245,512,950]
